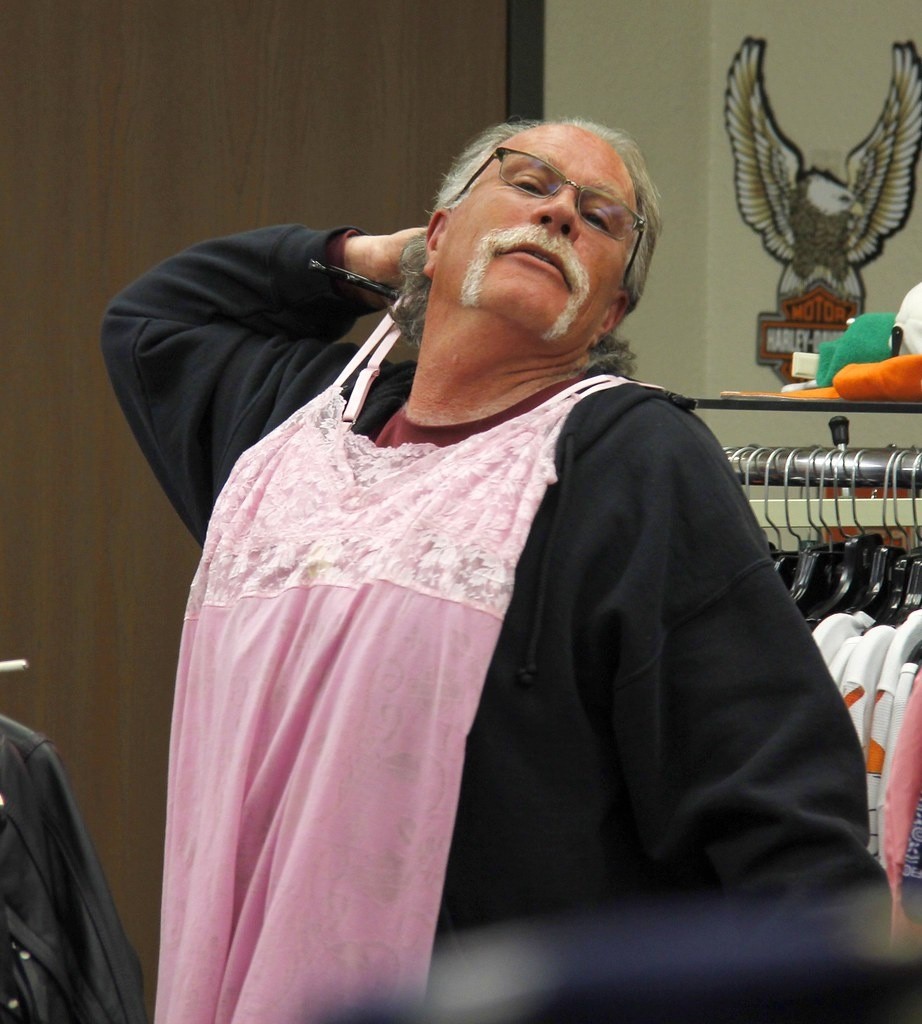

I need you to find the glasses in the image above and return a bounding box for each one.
[445,147,648,284]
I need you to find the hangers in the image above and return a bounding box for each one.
[719,443,922,664]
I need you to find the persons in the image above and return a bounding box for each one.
[99,119,891,1024]
